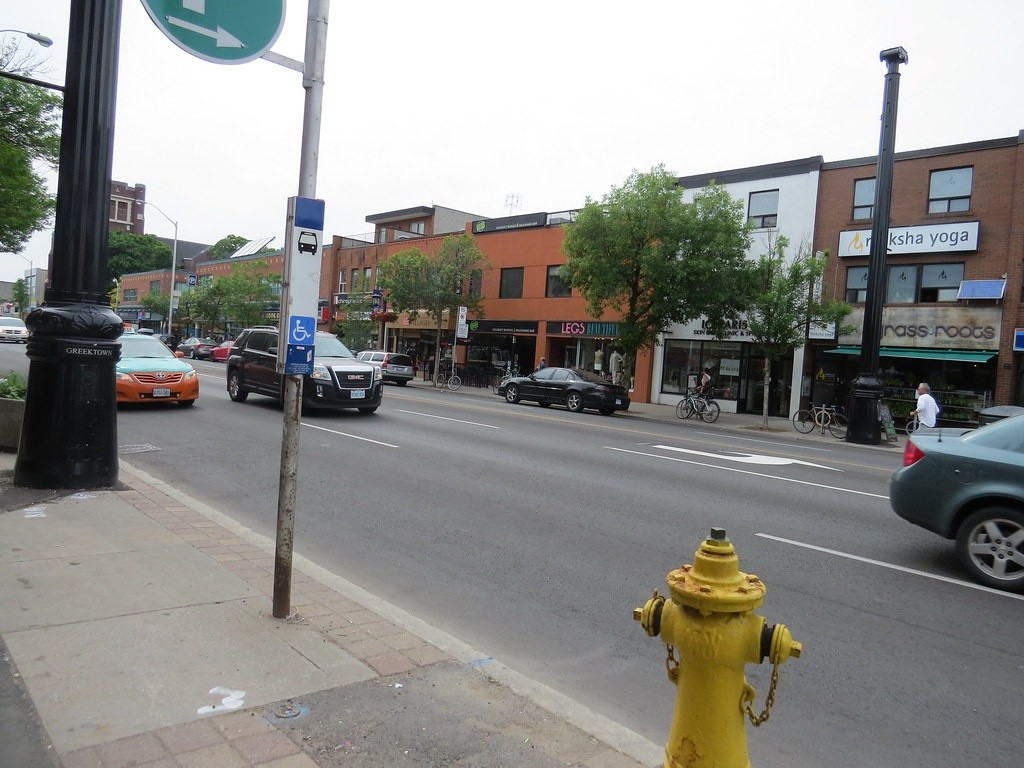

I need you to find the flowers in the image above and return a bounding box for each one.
[0,371,27,400]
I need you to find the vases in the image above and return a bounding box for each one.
[0,398,26,449]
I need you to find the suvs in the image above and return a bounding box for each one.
[356,350,415,387]
[226,325,383,416]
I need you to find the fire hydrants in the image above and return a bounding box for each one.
[633,526,800,768]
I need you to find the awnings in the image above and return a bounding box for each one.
[823,346,999,363]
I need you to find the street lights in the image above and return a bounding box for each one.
[112,277,119,315]
[135,198,178,334]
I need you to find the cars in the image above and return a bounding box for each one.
[889,411,1024,593]
[0,317,29,344]
[115,327,199,410]
[498,367,630,416]
[209,340,235,362]
[177,337,220,360]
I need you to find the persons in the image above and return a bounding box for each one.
[537,358,545,370]
[910,383,939,429]
[594,350,604,371]
[609,350,635,386]
[206,335,228,342]
[700,368,712,395]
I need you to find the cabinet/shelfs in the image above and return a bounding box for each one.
[882,387,984,431]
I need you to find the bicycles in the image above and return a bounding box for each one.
[492,366,506,394]
[793,401,849,439]
[675,386,720,423]
[435,367,461,391]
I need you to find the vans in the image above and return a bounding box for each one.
[151,333,177,353]
